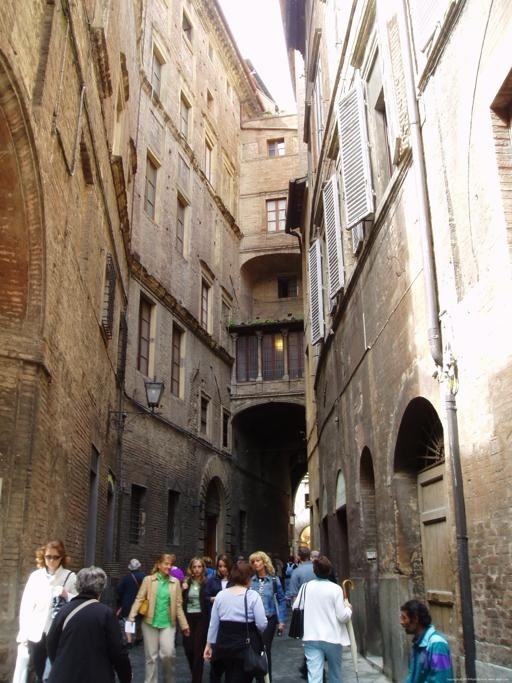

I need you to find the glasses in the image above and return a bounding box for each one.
[43,555,62,560]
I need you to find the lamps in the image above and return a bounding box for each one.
[109,375,165,415]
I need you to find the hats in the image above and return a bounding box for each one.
[128,559,141,571]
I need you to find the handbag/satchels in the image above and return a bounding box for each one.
[288,608,303,639]
[139,599,149,615]
[51,595,66,618]
[242,638,268,675]
[12,644,30,683]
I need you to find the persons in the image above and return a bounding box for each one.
[14,540,133,683]
[117,546,353,681]
[400,599,454,683]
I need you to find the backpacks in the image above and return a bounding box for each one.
[285,562,295,576]
